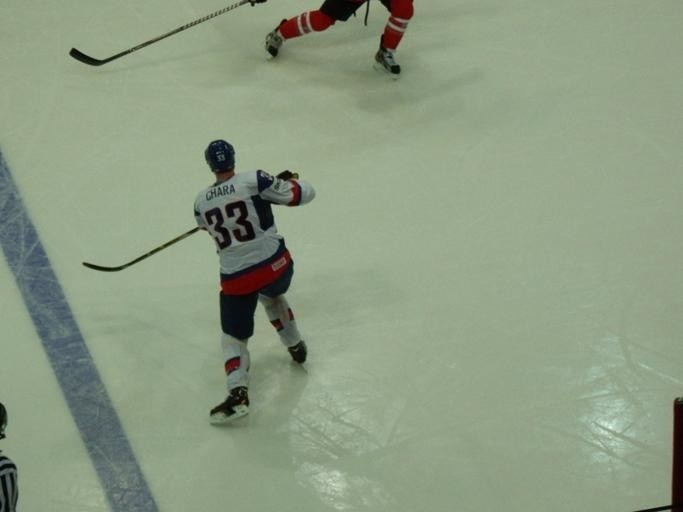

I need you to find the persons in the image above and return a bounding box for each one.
[193,140,316,425]
[1,403,19,512]
[263,1,414,80]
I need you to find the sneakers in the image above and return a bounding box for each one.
[209,389,248,418]
[288,340,308,364]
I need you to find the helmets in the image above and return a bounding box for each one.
[204,139,235,174]
